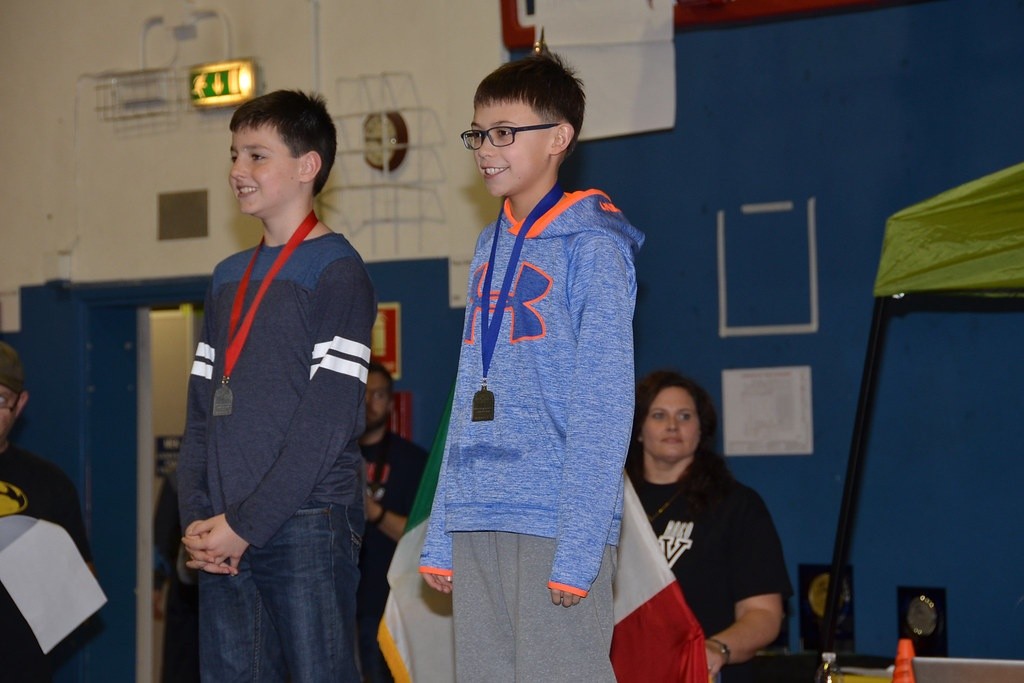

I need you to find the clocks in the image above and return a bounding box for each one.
[361,111,409,171]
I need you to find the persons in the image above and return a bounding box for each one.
[178,90,377,683]
[0,343,96,683]
[152,468,199,683]
[417,51,648,683]
[354,359,433,683]
[622,369,794,683]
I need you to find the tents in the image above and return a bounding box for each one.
[813,155,1024,663]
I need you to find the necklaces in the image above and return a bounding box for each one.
[635,481,690,523]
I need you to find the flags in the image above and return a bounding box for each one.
[378,357,710,683]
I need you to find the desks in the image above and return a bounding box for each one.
[719,651,894,683]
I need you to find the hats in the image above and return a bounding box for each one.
[0,341,24,392]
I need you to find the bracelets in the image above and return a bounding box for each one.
[707,639,730,667]
[370,506,387,524]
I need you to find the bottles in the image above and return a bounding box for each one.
[813,653,844,683]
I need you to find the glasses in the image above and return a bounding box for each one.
[461,123,560,150]
[0,394,21,416]
